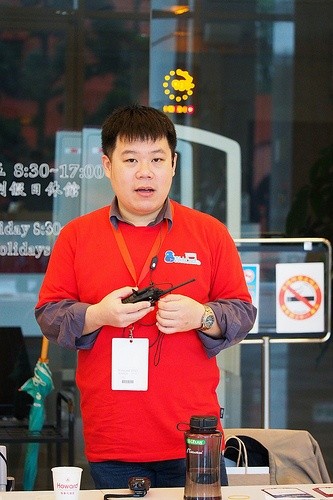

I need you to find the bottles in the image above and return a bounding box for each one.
[177,415,223,500]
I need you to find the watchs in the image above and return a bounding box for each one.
[197,305,214,331]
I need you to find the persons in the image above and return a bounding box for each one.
[35,105,257,489]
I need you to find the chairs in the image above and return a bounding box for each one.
[219,428,314,485]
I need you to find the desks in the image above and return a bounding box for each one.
[0,483,333,500]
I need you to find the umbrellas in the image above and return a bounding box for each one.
[18,336,55,491]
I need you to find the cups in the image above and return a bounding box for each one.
[51,466,83,500]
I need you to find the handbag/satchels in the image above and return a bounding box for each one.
[222,436,270,485]
